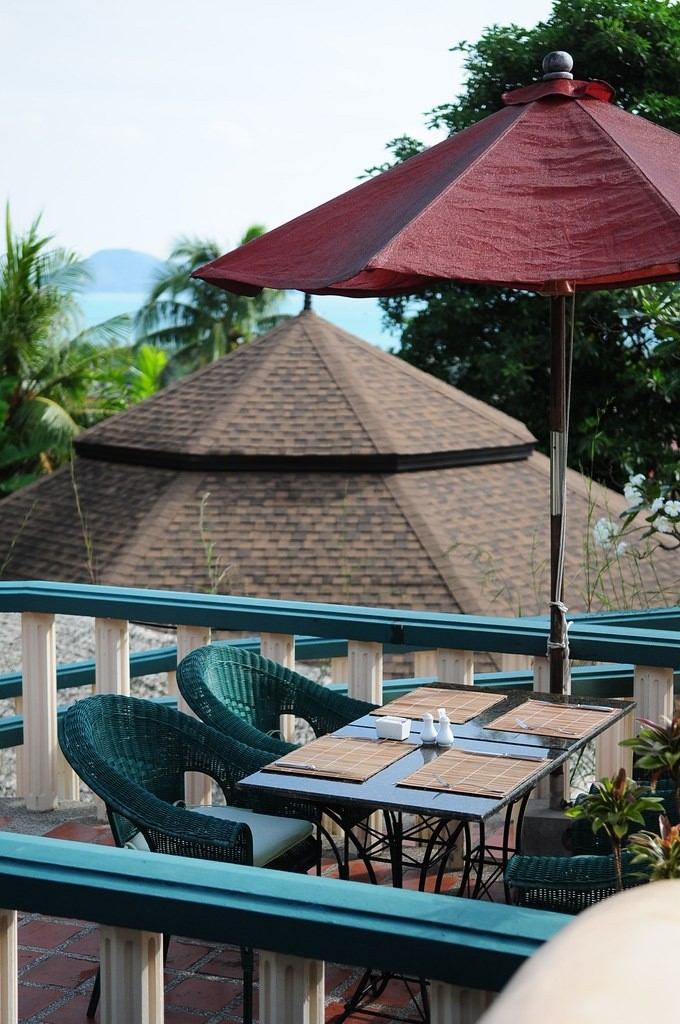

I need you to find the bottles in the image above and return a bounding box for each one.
[421,714,437,743]
[438,716,455,747]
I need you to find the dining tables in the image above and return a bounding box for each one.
[234,680,638,1024]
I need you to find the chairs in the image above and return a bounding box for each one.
[505,788,680,911]
[57,693,322,1024]
[176,644,384,885]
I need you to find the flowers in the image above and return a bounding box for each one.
[590,473,680,563]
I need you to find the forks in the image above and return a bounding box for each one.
[331,735,389,744]
[433,773,506,793]
[515,718,576,735]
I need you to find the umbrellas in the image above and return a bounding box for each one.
[189,49,680,696]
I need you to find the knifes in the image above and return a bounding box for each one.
[539,700,613,712]
[275,762,346,775]
[465,750,542,761]
